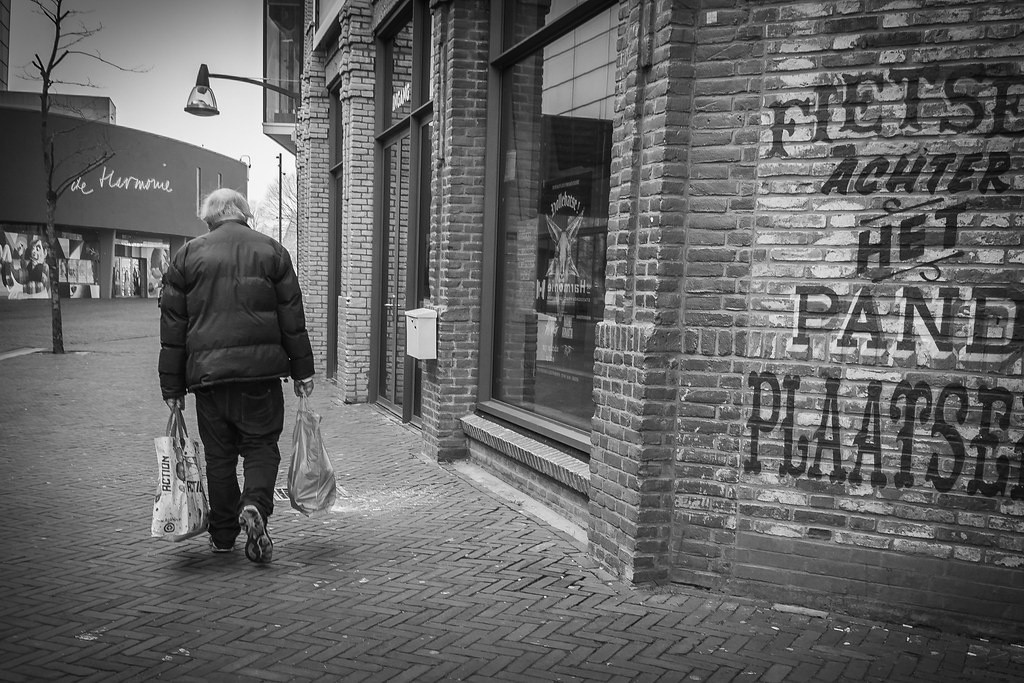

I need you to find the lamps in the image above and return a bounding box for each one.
[184,63,300,116]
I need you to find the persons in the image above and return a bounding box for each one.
[157,188,316,564]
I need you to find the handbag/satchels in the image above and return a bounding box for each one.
[287,384,336,520]
[151,403,209,542]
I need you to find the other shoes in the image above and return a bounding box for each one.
[209,534,233,552]
[239,505,274,564]
